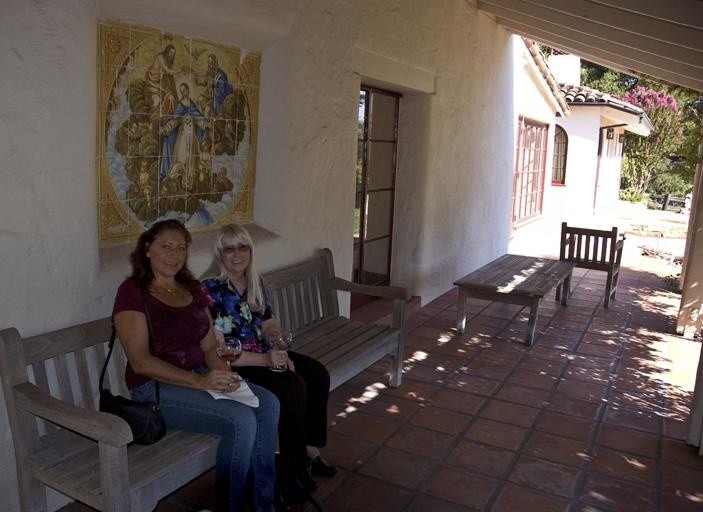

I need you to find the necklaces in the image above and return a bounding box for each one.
[167,289,175,296]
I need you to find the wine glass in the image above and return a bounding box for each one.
[267,330,292,372]
[216,337,242,374]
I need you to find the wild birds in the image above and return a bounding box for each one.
[183,44,209,62]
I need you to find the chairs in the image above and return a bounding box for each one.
[556,221,624,309]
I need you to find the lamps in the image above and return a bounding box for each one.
[619,133,626,144]
[606,128,614,140]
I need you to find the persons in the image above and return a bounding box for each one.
[199,223,338,496]
[143,43,234,204]
[111,219,280,512]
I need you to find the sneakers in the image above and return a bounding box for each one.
[294,455,337,490]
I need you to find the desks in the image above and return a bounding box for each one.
[453,253,577,346]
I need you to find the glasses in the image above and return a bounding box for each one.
[223,245,248,253]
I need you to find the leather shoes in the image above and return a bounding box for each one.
[98,389,165,446]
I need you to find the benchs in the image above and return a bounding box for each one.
[0,247,408,512]
